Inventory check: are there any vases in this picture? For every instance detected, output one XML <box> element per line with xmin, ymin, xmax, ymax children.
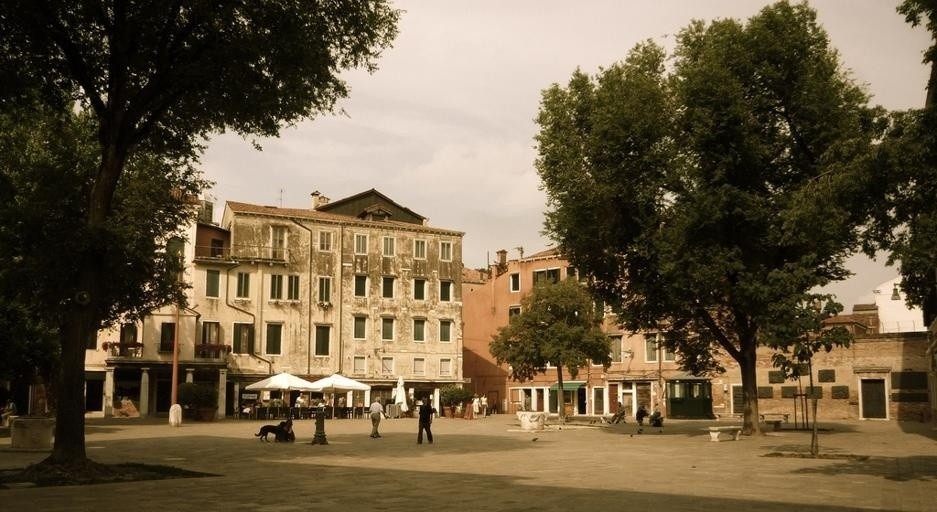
<box><xmin>515</xmin><ymin>409</ymin><xmax>547</xmax><ymax>430</ymax></box>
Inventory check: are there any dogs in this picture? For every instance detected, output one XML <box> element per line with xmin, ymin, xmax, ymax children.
<box><xmin>253</xmin><ymin>421</ymin><xmax>289</xmax><ymax>442</ymax></box>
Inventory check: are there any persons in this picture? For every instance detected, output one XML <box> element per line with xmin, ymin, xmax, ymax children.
<box><xmin>649</xmin><ymin>403</ymin><xmax>661</xmax><ymax>424</ymax></box>
<box><xmin>471</xmin><ymin>392</ymin><xmax>481</xmax><ymax>419</ymax></box>
<box><xmin>294</xmin><ymin>392</ymin><xmax>309</xmax><ymax>408</ymax></box>
<box><xmin>607</xmin><ymin>402</ymin><xmax>625</xmax><ymax>424</ymax></box>
<box><xmin>368</xmin><ymin>396</ymin><xmax>391</xmax><ymax>438</ymax></box>
<box><xmin>491</xmin><ymin>398</ymin><xmax>498</xmax><ymax>415</ymax></box>
<box><xmin>416</xmin><ymin>393</ymin><xmax>434</xmax><ymax>445</ymax></box>
<box><xmin>338</xmin><ymin>394</ymin><xmax>346</xmax><ymax>408</ymax></box>
<box><xmin>0</xmin><ymin>397</ymin><xmax>19</xmax><ymax>421</ymax></box>
<box><xmin>480</xmin><ymin>392</ymin><xmax>489</xmax><ymax>418</ymax></box>
<box><xmin>636</xmin><ymin>405</ymin><xmax>649</xmax><ymax>426</ymax></box>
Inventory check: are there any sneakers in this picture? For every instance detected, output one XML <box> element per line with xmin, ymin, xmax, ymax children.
<box><xmin>370</xmin><ymin>435</ymin><xmax>382</xmax><ymax>438</ymax></box>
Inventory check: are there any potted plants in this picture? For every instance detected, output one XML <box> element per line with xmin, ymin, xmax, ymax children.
<box><xmin>179</xmin><ymin>382</ymin><xmax>217</xmax><ymax>420</ymax></box>
<box><xmin>439</xmin><ymin>384</ymin><xmax>473</xmax><ymax>417</ymax></box>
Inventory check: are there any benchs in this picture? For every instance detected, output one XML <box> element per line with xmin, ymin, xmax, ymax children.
<box><xmin>708</xmin><ymin>426</ymin><xmax>743</xmax><ymax>440</ymax></box>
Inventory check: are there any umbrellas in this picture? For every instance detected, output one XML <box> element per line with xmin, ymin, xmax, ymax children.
<box><xmin>299</xmin><ymin>372</ymin><xmax>373</xmax><ymax>407</ymax></box>
<box><xmin>244</xmin><ymin>371</ymin><xmax>323</xmax><ymax>408</ymax></box>
<box><xmin>395</xmin><ymin>375</ymin><xmax>410</xmax><ymax>413</ymax></box>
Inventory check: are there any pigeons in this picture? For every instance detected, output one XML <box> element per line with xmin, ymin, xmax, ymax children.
<box><xmin>639</xmin><ymin>428</ymin><xmax>643</xmax><ymax>431</ymax></box>
<box><xmin>637</xmin><ymin>430</ymin><xmax>641</xmax><ymax>434</ymax></box>
<box><xmin>531</xmin><ymin>437</ymin><xmax>539</xmax><ymax>442</ymax></box>
